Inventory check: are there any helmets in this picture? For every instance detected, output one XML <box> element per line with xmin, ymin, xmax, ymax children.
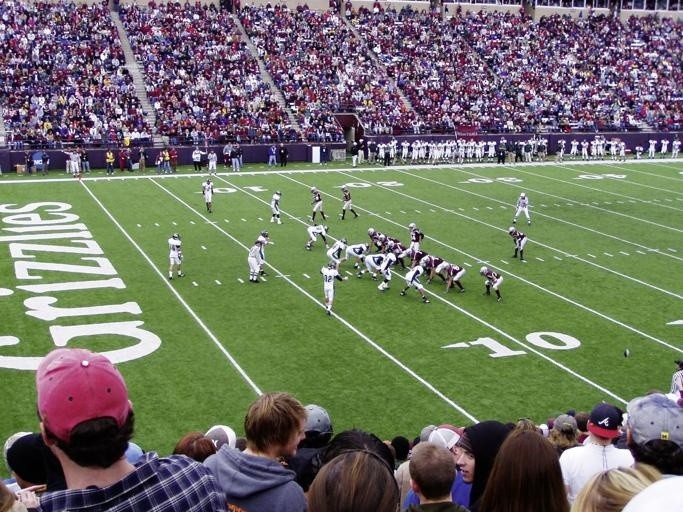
<box><xmin>479</xmin><ymin>266</ymin><xmax>488</xmax><ymax>274</ymax></box>
<box><xmin>255</xmin><ymin>230</ymin><xmax>269</xmax><ymax>248</ymax></box>
<box><xmin>341</xmin><ymin>185</ymin><xmax>347</xmax><ymax>191</ymax></box>
<box><xmin>520</xmin><ymin>193</ymin><xmax>526</xmax><ymax>197</ymax></box>
<box><xmin>311</xmin><ymin>186</ymin><xmax>318</xmax><ymax>194</ymax></box>
<box><xmin>276</xmin><ymin>191</ymin><xmax>282</xmax><ymax>195</ymax></box>
<box><xmin>320</xmin><ymin>221</ymin><xmax>451</xmax><ymax>271</ymax></box>
<box><xmin>207</xmin><ymin>179</ymin><xmax>212</xmax><ymax>183</ymax></box>
<box><xmin>508</xmin><ymin>226</ymin><xmax>516</xmax><ymax>233</ymax></box>
<box><xmin>172</xmin><ymin>233</ymin><xmax>179</xmax><ymax>239</ymax></box>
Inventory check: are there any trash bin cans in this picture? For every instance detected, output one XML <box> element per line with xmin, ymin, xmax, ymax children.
<box><xmin>306</xmin><ymin>144</ymin><xmax>321</xmax><ymax>163</ymax></box>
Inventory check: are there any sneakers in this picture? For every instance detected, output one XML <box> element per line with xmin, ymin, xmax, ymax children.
<box><xmin>270</xmin><ymin>217</ymin><xmax>283</xmax><ymax>225</ymax></box>
<box><xmin>248</xmin><ymin>270</ymin><xmax>267</xmax><ymax>283</ymax></box>
<box><xmin>352</xmin><ymin>260</ymin><xmax>503</xmax><ymax>303</ymax></box>
<box><xmin>324</xmin><ymin>304</ymin><xmax>334</xmax><ymax>315</ymax></box>
<box><xmin>168</xmin><ymin>271</ymin><xmax>185</xmax><ymax>280</ymax></box>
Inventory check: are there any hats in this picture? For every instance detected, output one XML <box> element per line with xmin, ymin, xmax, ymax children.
<box><xmin>627</xmin><ymin>393</ymin><xmax>683</xmax><ymax>456</ymax></box>
<box><xmin>540</xmin><ymin>402</ymin><xmax>623</xmax><ymax>440</ymax></box>
<box><xmin>203</xmin><ymin>425</ymin><xmax>236</xmax><ymax>453</ymax></box>
<box><xmin>419</xmin><ymin>424</ymin><xmax>471</xmax><ymax>455</ymax></box>
<box><xmin>32</xmin><ymin>342</ymin><xmax>133</xmax><ymax>441</ymax></box>
<box><xmin>303</xmin><ymin>404</ymin><xmax>331</xmax><ymax>436</ymax></box>
<box><xmin>3</xmin><ymin>431</ymin><xmax>67</xmax><ymax>492</ymax></box>
